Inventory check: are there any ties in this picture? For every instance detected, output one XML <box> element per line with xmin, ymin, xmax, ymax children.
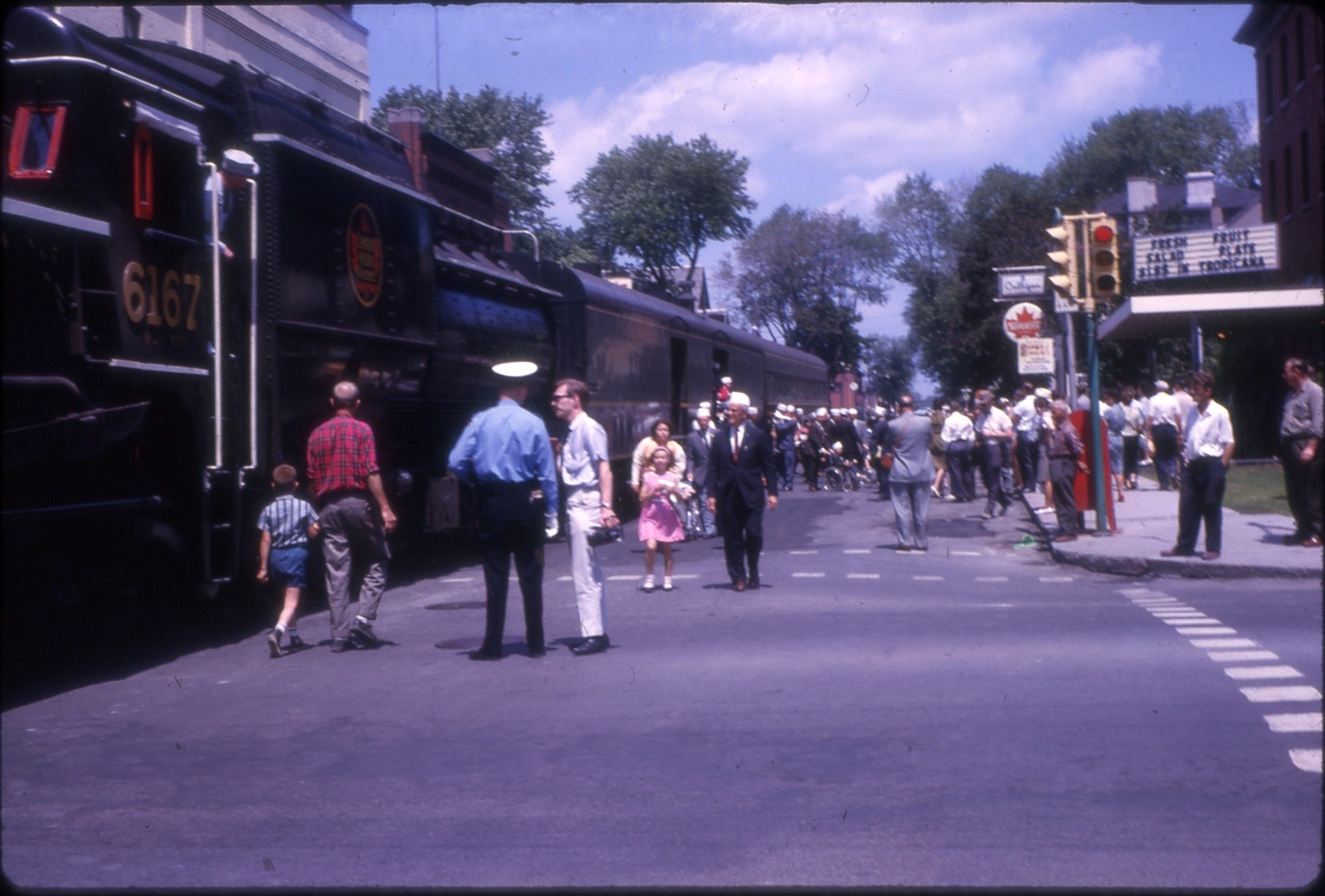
<box><xmin>732</xmin><ymin>423</ymin><xmax>740</xmax><ymax>463</ymax></box>
<box><xmin>559</xmin><ymin>428</ymin><xmax>570</xmax><ymax>445</ymax></box>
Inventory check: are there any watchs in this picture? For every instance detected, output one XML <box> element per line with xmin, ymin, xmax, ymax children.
<box><xmin>599</xmin><ymin>500</ymin><xmax>613</xmax><ymax>509</ymax></box>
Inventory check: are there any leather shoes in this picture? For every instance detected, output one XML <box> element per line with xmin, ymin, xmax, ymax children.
<box><xmin>734</xmin><ymin>581</ymin><xmax>746</xmax><ymax>591</ymax></box>
<box><xmin>1284</xmin><ymin>532</ymin><xmax>1325</xmax><ymax>547</ymax></box>
<box><xmin>529</xmin><ymin>646</ymin><xmax>547</xmax><ymax>658</ymax></box>
<box><xmin>670</xmin><ymin>453</ymin><xmax>1186</xmax><ymax>554</ymax></box>
<box><xmin>643</xmin><ymin>585</ymin><xmax>656</xmax><ymax>593</ymax></box>
<box><xmin>1201</xmin><ymin>550</ymin><xmax>1220</xmax><ymax>561</ymax></box>
<box><xmin>468</xmin><ymin>648</ymin><xmax>501</xmax><ymax>662</ymax></box>
<box><xmin>350</xmin><ymin>618</ymin><xmax>382</xmax><ymax>645</ymax></box>
<box><xmin>662</xmin><ymin>584</ymin><xmax>673</xmax><ymax>591</ymax></box>
<box><xmin>569</xmin><ymin>633</ymin><xmax>610</xmax><ymax>655</ymax></box>
<box><xmin>331</xmin><ymin>638</ymin><xmax>358</xmax><ymax>653</ymax></box>
<box><xmin>748</xmin><ymin>571</ymin><xmax>760</xmax><ymax>588</ymax></box>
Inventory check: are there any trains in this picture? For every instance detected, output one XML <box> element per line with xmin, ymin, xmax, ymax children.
<box><xmin>0</xmin><ymin>0</ymin><xmax>829</xmax><ymax>603</ymax></box>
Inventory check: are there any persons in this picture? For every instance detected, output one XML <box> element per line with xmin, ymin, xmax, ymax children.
<box><xmin>1274</xmin><ymin>358</ymin><xmax>1324</xmax><ymax>547</ymax></box>
<box><xmin>305</xmin><ymin>381</ymin><xmax>399</xmax><ymax>652</ymax></box>
<box><xmin>547</xmin><ymin>378</ymin><xmax>621</xmax><ymax>655</ymax></box>
<box><xmin>257</xmin><ymin>465</ymin><xmax>321</xmax><ymax>657</ymax></box>
<box><xmin>626</xmin><ymin>374</ymin><xmax>1236</xmax><ymax>591</ymax></box>
<box><xmin>446</xmin><ymin>362</ymin><xmax>558</xmax><ymax>658</ymax></box>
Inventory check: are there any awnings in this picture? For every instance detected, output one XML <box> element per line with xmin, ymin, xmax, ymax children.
<box><xmin>1096</xmin><ymin>288</ymin><xmax>1325</xmax><ymax>376</ymax></box>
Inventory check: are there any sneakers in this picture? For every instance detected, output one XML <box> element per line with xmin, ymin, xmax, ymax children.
<box><xmin>289</xmin><ymin>635</ymin><xmax>309</xmax><ymax>654</ymax></box>
<box><xmin>267</xmin><ymin>629</ymin><xmax>284</xmax><ymax>658</ymax></box>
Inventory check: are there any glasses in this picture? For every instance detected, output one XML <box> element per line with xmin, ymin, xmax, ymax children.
<box><xmin>551</xmin><ymin>393</ymin><xmax>572</xmax><ymax>401</ymax></box>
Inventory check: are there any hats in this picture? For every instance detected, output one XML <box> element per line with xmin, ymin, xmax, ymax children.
<box><xmin>1154</xmin><ymin>379</ymin><xmax>1171</xmax><ymax>391</ymax></box>
<box><xmin>645</xmin><ymin>378</ymin><xmax>1066</xmax><ymax>423</ymax></box>
<box><xmin>489</xmin><ymin>361</ymin><xmax>541</xmax><ymax>387</ymax></box>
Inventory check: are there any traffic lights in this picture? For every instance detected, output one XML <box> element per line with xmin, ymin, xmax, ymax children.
<box><xmin>1044</xmin><ymin>224</ymin><xmax>1077</xmax><ymax>305</ymax></box>
<box><xmin>1089</xmin><ymin>219</ymin><xmax>1120</xmax><ymax>298</ymax></box>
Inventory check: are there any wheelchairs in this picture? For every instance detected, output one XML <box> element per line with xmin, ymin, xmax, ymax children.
<box><xmin>824</xmin><ymin>452</ymin><xmax>858</xmax><ymax>493</ymax></box>
